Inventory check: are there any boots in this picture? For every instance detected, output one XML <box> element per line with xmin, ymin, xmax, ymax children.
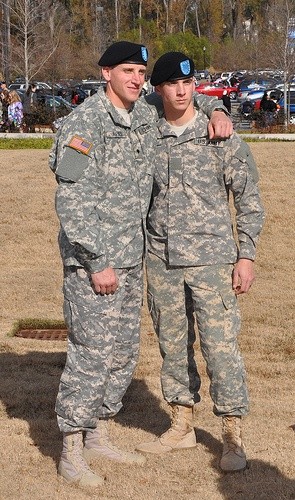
<box><xmin>84</xmin><ymin>419</ymin><xmax>147</xmax><ymax>466</ymax></box>
<box><xmin>57</xmin><ymin>430</ymin><xmax>105</xmax><ymax>490</ymax></box>
<box><xmin>134</xmin><ymin>404</ymin><xmax>197</xmax><ymax>454</ymax></box>
<box><xmin>221</xmin><ymin>415</ymin><xmax>247</xmax><ymax>470</ymax></box>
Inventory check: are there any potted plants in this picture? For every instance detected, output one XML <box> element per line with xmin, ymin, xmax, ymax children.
<box><xmin>22</xmin><ymin>103</ymin><xmax>57</xmax><ymax>133</ymax></box>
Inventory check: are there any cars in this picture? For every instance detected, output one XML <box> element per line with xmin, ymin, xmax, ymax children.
<box><xmin>0</xmin><ymin>77</ymin><xmax>106</xmax><ymax>125</ymax></box>
<box><xmin>193</xmin><ymin>66</ymin><xmax>295</xmax><ymax>125</ymax></box>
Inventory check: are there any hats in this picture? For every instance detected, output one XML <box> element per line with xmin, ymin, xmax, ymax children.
<box><xmin>150</xmin><ymin>52</ymin><xmax>196</xmax><ymax>86</ymax></box>
<box><xmin>97</xmin><ymin>40</ymin><xmax>148</xmax><ymax>67</ymax></box>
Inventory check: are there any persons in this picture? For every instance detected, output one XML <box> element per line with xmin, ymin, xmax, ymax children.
<box><xmin>49</xmin><ymin>41</ymin><xmax>232</xmax><ymax>488</ymax></box>
<box><xmin>0</xmin><ymin>79</ymin><xmax>38</xmax><ymax>133</ymax></box>
<box><xmin>134</xmin><ymin>53</ymin><xmax>264</xmax><ymax>471</ymax></box>
